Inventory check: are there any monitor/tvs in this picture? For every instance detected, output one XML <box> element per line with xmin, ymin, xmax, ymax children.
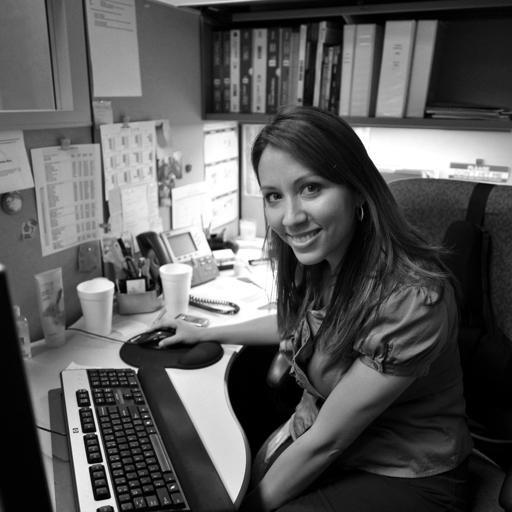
<box><xmin>0</xmin><ymin>266</ymin><xmax>53</xmax><ymax>512</ymax></box>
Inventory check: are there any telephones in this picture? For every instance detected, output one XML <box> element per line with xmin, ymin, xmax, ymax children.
<box><xmin>136</xmin><ymin>227</ymin><xmax>219</xmax><ymax>288</ymax></box>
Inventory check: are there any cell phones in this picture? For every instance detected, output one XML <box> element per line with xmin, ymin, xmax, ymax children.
<box><xmin>175</xmin><ymin>313</ymin><xmax>208</xmax><ymax>327</ymax></box>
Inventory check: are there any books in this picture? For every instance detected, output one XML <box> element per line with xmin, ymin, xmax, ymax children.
<box><xmin>209</xmin><ymin>17</ymin><xmax>439</xmax><ymax>119</ymax></box>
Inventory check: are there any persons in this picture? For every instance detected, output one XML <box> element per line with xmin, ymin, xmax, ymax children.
<box><xmin>143</xmin><ymin>104</ymin><xmax>482</xmax><ymax>509</ymax></box>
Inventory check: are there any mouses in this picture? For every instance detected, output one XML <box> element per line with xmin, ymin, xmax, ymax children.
<box><xmin>138</xmin><ymin>327</ymin><xmax>175</xmax><ymax>347</ymax></box>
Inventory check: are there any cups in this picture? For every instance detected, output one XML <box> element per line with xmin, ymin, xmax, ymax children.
<box><xmin>75</xmin><ymin>277</ymin><xmax>116</xmax><ymax>341</ymax></box>
<box><xmin>155</xmin><ymin>263</ymin><xmax>196</xmax><ymax>319</ymax></box>
<box><xmin>240</xmin><ymin>217</ymin><xmax>257</xmax><ymax>238</ymax></box>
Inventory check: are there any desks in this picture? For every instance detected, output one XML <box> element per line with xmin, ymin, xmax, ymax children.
<box><xmin>21</xmin><ymin>235</ymin><xmax>279</xmax><ymax>512</ymax></box>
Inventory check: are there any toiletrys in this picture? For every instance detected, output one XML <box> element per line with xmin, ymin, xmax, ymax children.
<box><xmin>34</xmin><ymin>266</ymin><xmax>66</xmax><ymax>349</ymax></box>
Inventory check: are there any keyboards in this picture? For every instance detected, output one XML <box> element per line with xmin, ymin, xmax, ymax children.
<box><xmin>60</xmin><ymin>369</ymin><xmax>190</xmax><ymax>512</ymax></box>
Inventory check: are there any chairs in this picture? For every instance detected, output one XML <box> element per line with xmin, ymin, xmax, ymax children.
<box><xmin>381</xmin><ymin>178</ymin><xmax>512</xmax><ymax>510</ymax></box>
